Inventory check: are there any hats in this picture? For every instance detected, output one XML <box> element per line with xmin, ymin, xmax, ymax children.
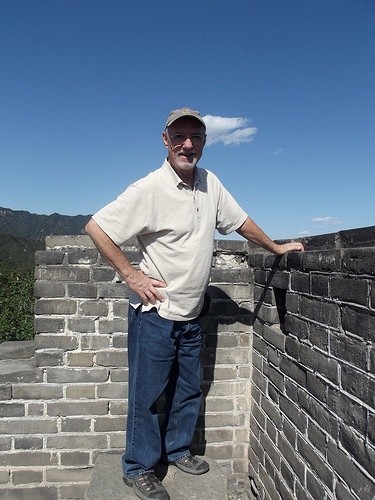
<box><xmin>163</xmin><ymin>107</ymin><xmax>208</xmax><ymax>130</ymax></box>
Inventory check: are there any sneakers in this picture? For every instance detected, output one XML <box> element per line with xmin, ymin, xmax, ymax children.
<box><xmin>169</xmin><ymin>453</ymin><xmax>210</xmax><ymax>475</ymax></box>
<box><xmin>122</xmin><ymin>469</ymin><xmax>173</xmax><ymax>500</ymax></box>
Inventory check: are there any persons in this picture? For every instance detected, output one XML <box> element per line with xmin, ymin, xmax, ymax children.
<box><xmin>84</xmin><ymin>107</ymin><xmax>305</xmax><ymax>500</ymax></box>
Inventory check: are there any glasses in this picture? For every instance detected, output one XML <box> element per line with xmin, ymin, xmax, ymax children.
<box><xmin>167</xmin><ymin>132</ymin><xmax>207</xmax><ymax>144</ymax></box>
